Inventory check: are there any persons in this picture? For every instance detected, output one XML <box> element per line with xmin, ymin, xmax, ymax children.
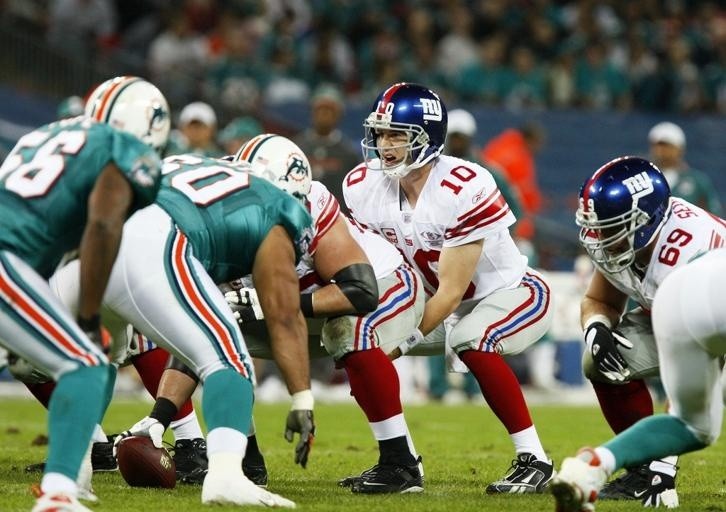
<box><xmin>0</xmin><ymin>74</ymin><xmax>169</xmax><ymax>511</ymax></box>
<box><xmin>1</xmin><ymin>1</ymin><xmax>725</xmax><ymax>404</ymax></box>
<box><xmin>551</xmin><ymin>155</ymin><xmax>725</xmax><ymax>511</ymax></box>
<box><xmin>46</xmin><ymin>84</ymin><xmax>553</xmax><ymax>508</ymax></box>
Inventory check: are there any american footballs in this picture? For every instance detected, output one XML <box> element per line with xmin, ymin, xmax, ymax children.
<box><xmin>116</xmin><ymin>436</ymin><xmax>176</xmax><ymax>487</ymax></box>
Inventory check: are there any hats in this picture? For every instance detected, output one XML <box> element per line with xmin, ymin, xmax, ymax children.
<box><xmin>176</xmin><ymin>101</ymin><xmax>217</xmax><ymax>128</ymax></box>
<box><xmin>445</xmin><ymin>108</ymin><xmax>476</xmax><ymax>138</ymax></box>
<box><xmin>648</xmin><ymin>121</ymin><xmax>686</xmax><ymax>149</ymax></box>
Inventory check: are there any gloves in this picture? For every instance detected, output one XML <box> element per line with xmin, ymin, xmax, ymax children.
<box><xmin>76</xmin><ymin>312</ymin><xmax>104</xmax><ymax>354</ymax></box>
<box><xmin>583</xmin><ymin>321</ymin><xmax>633</xmax><ymax>382</ymax></box>
<box><xmin>113</xmin><ymin>416</ymin><xmax>165</xmax><ymax>456</ymax></box>
<box><xmin>284</xmin><ymin>389</ymin><xmax>315</xmax><ymax>467</ymax></box>
<box><xmin>632</xmin><ymin>460</ymin><xmax>680</xmax><ymax>509</ymax></box>
<box><xmin>223</xmin><ymin>286</ymin><xmax>265</xmax><ymax>325</ymax></box>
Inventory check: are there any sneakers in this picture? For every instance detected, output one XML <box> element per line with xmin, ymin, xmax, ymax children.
<box><xmin>485</xmin><ymin>453</ymin><xmax>557</xmax><ymax>494</ymax></box>
<box><xmin>23</xmin><ymin>435</ymin><xmax>297</xmax><ymax>512</ymax></box>
<box><xmin>350</xmin><ymin>454</ymin><xmax>424</xmax><ymax>495</ymax></box>
<box><xmin>597</xmin><ymin>465</ymin><xmax>648</xmax><ymax>500</ymax></box>
<box><xmin>548</xmin><ymin>447</ymin><xmax>608</xmax><ymax>512</ymax></box>
<box><xmin>336</xmin><ymin>452</ymin><xmax>425</xmax><ymax>488</ymax></box>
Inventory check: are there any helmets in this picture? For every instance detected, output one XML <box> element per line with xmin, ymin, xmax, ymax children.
<box><xmin>83</xmin><ymin>74</ymin><xmax>171</xmax><ymax>157</ymax></box>
<box><xmin>230</xmin><ymin>132</ymin><xmax>312</xmax><ymax>198</ymax></box>
<box><xmin>359</xmin><ymin>82</ymin><xmax>448</xmax><ymax>182</ymax></box>
<box><xmin>574</xmin><ymin>155</ymin><xmax>672</xmax><ymax>275</ymax></box>
